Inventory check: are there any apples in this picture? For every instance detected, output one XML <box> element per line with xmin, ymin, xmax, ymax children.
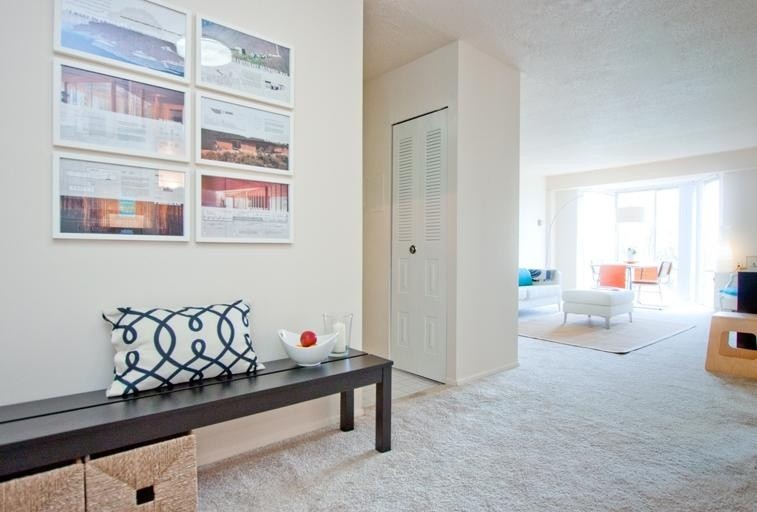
<box><xmin>300</xmin><ymin>332</ymin><xmax>316</xmax><ymax>347</ymax></box>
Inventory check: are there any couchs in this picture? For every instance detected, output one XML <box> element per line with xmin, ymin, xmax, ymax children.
<box><xmin>517</xmin><ymin>265</ymin><xmax>567</xmax><ymax>311</ymax></box>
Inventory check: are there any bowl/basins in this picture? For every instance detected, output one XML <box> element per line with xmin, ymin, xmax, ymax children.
<box><xmin>278</xmin><ymin>329</ymin><xmax>339</xmax><ymax>366</ymax></box>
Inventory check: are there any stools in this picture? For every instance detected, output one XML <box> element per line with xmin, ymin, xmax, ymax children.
<box><xmin>704</xmin><ymin>310</ymin><xmax>757</xmax><ymax>380</ymax></box>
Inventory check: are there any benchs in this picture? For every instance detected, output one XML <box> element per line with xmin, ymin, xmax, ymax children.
<box><xmin>0</xmin><ymin>340</ymin><xmax>394</xmax><ymax>479</ymax></box>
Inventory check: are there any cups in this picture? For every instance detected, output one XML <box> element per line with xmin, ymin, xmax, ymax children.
<box><xmin>321</xmin><ymin>312</ymin><xmax>353</xmax><ymax>357</ymax></box>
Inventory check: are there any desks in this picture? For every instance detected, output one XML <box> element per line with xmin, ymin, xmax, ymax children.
<box><xmin>561</xmin><ymin>282</ymin><xmax>636</xmax><ymax>330</ymax></box>
<box><xmin>736</xmin><ymin>270</ymin><xmax>757</xmax><ymax>312</ymax></box>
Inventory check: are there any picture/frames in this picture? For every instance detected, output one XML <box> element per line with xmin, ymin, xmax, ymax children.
<box><xmin>52</xmin><ymin>59</ymin><xmax>192</xmax><ymax>164</ymax></box>
<box><xmin>194</xmin><ymin>91</ymin><xmax>293</xmax><ymax>175</ymax></box>
<box><xmin>51</xmin><ymin>152</ymin><xmax>190</xmax><ymax>242</ymax></box>
<box><xmin>194</xmin><ymin>170</ymin><xmax>293</xmax><ymax>244</ymax></box>
<box><xmin>194</xmin><ymin>14</ymin><xmax>293</xmax><ymax>108</ymax></box>
<box><xmin>50</xmin><ymin>1</ymin><xmax>192</xmax><ymax>85</ymax></box>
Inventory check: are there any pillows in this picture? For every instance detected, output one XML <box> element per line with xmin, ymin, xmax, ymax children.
<box><xmin>517</xmin><ymin>268</ymin><xmax>557</xmax><ymax>288</ymax></box>
<box><xmin>100</xmin><ymin>299</ymin><xmax>267</xmax><ymax>398</ymax></box>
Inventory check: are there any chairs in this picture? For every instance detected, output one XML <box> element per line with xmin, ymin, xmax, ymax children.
<box><xmin>586</xmin><ymin>257</ymin><xmax>673</xmax><ymax>311</ymax></box>
